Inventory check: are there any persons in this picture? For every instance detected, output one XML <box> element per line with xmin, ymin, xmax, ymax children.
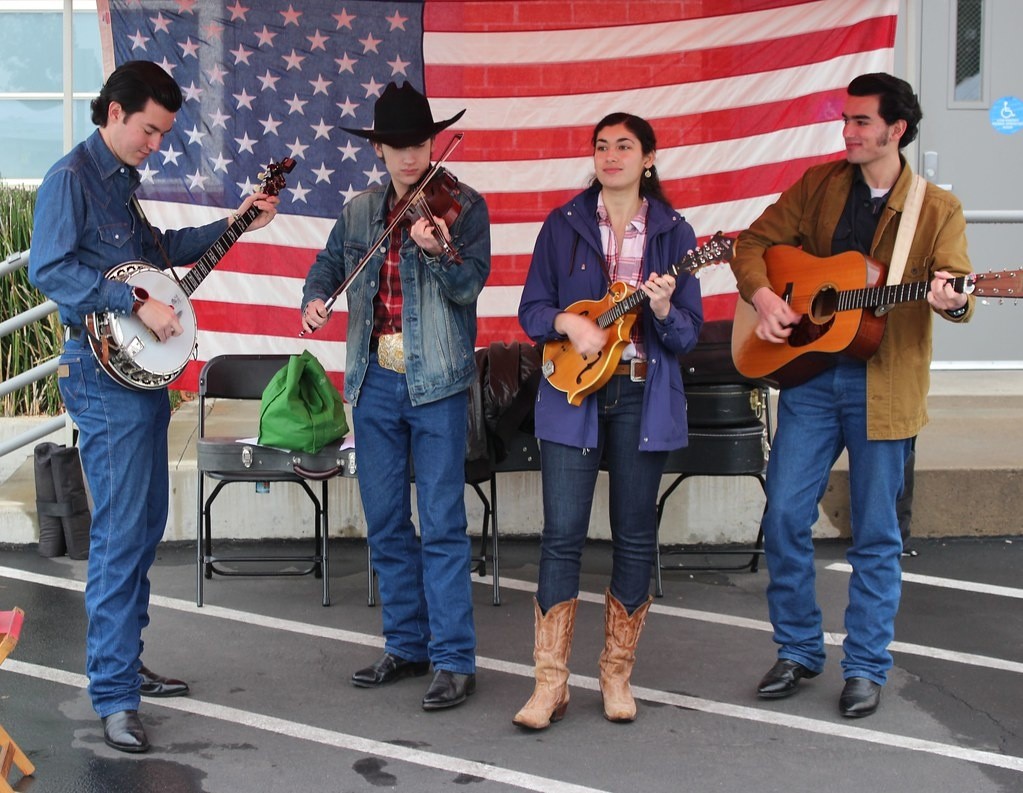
<box><xmin>512</xmin><ymin>111</ymin><xmax>704</xmax><ymax>730</ymax></box>
<box><xmin>27</xmin><ymin>60</ymin><xmax>281</xmax><ymax>751</ymax></box>
<box><xmin>299</xmin><ymin>80</ymin><xmax>492</xmax><ymax>710</ymax></box>
<box><xmin>726</xmin><ymin>72</ymin><xmax>977</xmax><ymax>719</ymax></box>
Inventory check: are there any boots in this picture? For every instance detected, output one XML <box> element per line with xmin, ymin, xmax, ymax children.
<box><xmin>599</xmin><ymin>584</ymin><xmax>653</xmax><ymax>722</ymax></box>
<box><xmin>513</xmin><ymin>589</ymin><xmax>578</xmax><ymax>730</ymax></box>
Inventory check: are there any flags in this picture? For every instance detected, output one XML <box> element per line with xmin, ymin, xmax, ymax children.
<box><xmin>108</xmin><ymin>0</ymin><xmax>900</xmax><ymax>403</ymax></box>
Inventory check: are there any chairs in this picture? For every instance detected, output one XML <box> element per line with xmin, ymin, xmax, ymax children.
<box><xmin>345</xmin><ymin>319</ymin><xmax>774</xmax><ymax>607</ymax></box>
<box><xmin>196</xmin><ymin>354</ymin><xmax>330</xmax><ymax>607</ymax></box>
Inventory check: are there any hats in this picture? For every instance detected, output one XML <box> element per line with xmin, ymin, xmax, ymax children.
<box><xmin>339</xmin><ymin>80</ymin><xmax>468</xmax><ymax>150</ymax></box>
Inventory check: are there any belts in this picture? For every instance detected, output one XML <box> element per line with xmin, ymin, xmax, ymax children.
<box><xmin>369</xmin><ymin>333</ymin><xmax>409</xmax><ymax>374</ymax></box>
<box><xmin>609</xmin><ymin>360</ymin><xmax>652</xmax><ymax>382</ymax></box>
<box><xmin>68</xmin><ymin>326</ymin><xmax>92</xmax><ymax>346</ymax></box>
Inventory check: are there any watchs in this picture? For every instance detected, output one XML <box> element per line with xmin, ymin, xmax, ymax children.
<box><xmin>132</xmin><ymin>287</ymin><xmax>149</xmax><ymax>313</ymax></box>
<box><xmin>946</xmin><ymin>306</ymin><xmax>967</xmax><ymax>317</ymax></box>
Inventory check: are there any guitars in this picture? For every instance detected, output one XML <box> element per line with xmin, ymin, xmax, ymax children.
<box><xmin>85</xmin><ymin>156</ymin><xmax>297</xmax><ymax>391</ymax></box>
<box><xmin>542</xmin><ymin>230</ymin><xmax>738</xmax><ymax>407</ymax></box>
<box><xmin>731</xmin><ymin>244</ymin><xmax>1023</xmax><ymax>390</ymax></box>
<box><xmin>385</xmin><ymin>165</ymin><xmax>464</xmax><ymax>267</ymax></box>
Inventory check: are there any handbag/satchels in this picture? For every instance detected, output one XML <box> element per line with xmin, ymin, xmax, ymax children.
<box><xmin>256</xmin><ymin>350</ymin><xmax>349</xmax><ymax>455</ymax></box>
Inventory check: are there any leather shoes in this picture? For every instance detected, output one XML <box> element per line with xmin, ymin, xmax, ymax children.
<box><xmin>754</xmin><ymin>657</ymin><xmax>820</xmax><ymax>699</ymax></box>
<box><xmin>135</xmin><ymin>664</ymin><xmax>190</xmax><ymax>696</ymax></box>
<box><xmin>838</xmin><ymin>675</ymin><xmax>882</xmax><ymax>718</ymax></box>
<box><xmin>348</xmin><ymin>651</ymin><xmax>431</xmax><ymax>688</ymax></box>
<box><xmin>101</xmin><ymin>710</ymin><xmax>150</xmax><ymax>751</ymax></box>
<box><xmin>421</xmin><ymin>667</ymin><xmax>478</xmax><ymax>710</ymax></box>
